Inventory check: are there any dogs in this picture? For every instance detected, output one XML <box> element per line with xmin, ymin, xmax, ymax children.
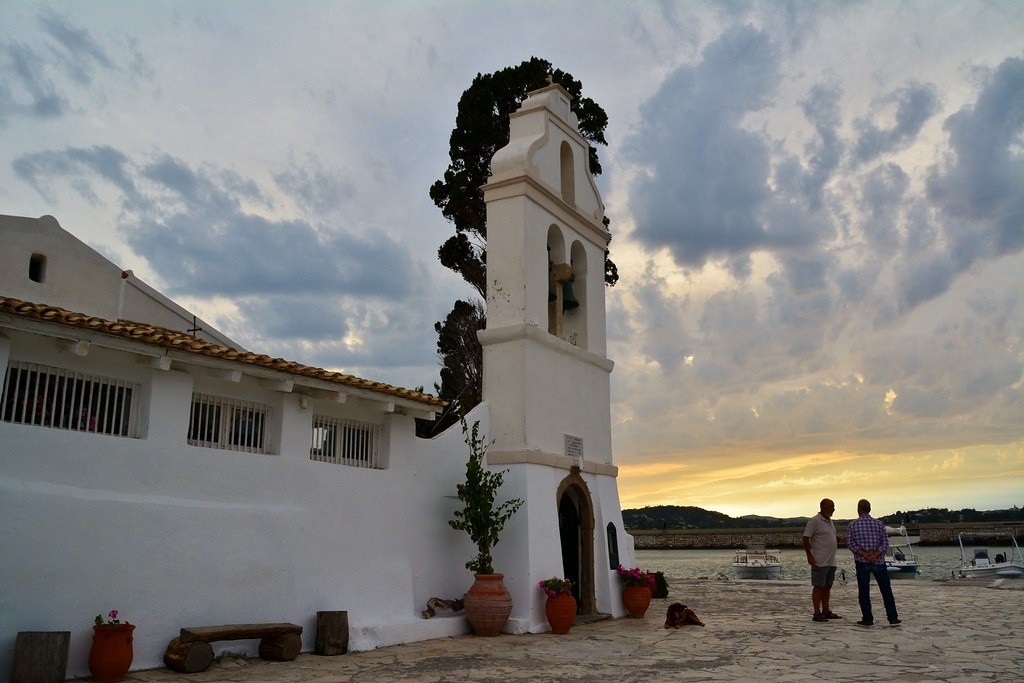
<box><xmin>663</xmin><ymin>602</ymin><xmax>704</xmax><ymax>629</ymax></box>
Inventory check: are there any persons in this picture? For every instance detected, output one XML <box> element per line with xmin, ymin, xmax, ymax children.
<box><xmin>847</xmin><ymin>499</ymin><xmax>902</xmax><ymax>626</ymax></box>
<box><xmin>802</xmin><ymin>499</ymin><xmax>842</xmax><ymax>622</ymax></box>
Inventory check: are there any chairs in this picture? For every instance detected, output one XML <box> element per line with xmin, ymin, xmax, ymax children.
<box><xmin>971</xmin><ymin>552</ymin><xmax>991</xmax><ymax>565</ymax></box>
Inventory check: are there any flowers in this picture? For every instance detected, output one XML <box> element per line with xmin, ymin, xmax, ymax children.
<box><xmin>616</xmin><ymin>563</ymin><xmax>655</xmax><ymax>590</ymax></box>
<box><xmin>94</xmin><ymin>610</ymin><xmax>130</xmax><ymax>625</ymax></box>
<box><xmin>537</xmin><ymin>576</ymin><xmax>575</xmax><ymax>597</ymax></box>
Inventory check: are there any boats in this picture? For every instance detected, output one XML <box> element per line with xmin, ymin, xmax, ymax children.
<box><xmin>952</xmin><ymin>528</ymin><xmax>1024</xmax><ymax>579</ymax></box>
<box><xmin>731</xmin><ymin>543</ymin><xmax>784</xmax><ymax>581</ymax></box>
<box><xmin>883</xmin><ymin>525</ymin><xmax>922</xmax><ymax>575</ymax></box>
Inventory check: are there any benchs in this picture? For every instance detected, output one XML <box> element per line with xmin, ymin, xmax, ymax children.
<box><xmin>163</xmin><ymin>623</ymin><xmax>303</xmax><ymax>674</ymax></box>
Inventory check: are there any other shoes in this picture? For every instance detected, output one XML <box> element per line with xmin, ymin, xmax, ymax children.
<box><xmin>857</xmin><ymin>617</ymin><xmax>874</xmax><ymax>626</ymax></box>
<box><xmin>821</xmin><ymin>610</ymin><xmax>842</xmax><ymax>619</ymax></box>
<box><xmin>812</xmin><ymin>613</ymin><xmax>828</xmax><ymax>622</ymax></box>
<box><xmin>887</xmin><ymin>618</ymin><xmax>902</xmax><ymax>626</ymax></box>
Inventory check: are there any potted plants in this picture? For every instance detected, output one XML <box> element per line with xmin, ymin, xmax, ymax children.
<box><xmin>448</xmin><ymin>396</ymin><xmax>525</xmax><ymax>635</ymax></box>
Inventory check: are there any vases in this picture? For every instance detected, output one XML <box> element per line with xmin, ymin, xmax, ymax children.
<box><xmin>624</xmin><ymin>584</ymin><xmax>652</xmax><ymax>619</ymax></box>
<box><xmin>89</xmin><ymin>624</ymin><xmax>135</xmax><ymax>683</ymax></box>
<box><xmin>545</xmin><ymin>590</ymin><xmax>578</xmax><ymax>634</ymax></box>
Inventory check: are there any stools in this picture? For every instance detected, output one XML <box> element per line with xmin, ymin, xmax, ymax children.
<box><xmin>314</xmin><ymin>611</ymin><xmax>349</xmax><ymax>656</ymax></box>
<box><xmin>11</xmin><ymin>630</ymin><xmax>70</xmax><ymax>683</ymax></box>
